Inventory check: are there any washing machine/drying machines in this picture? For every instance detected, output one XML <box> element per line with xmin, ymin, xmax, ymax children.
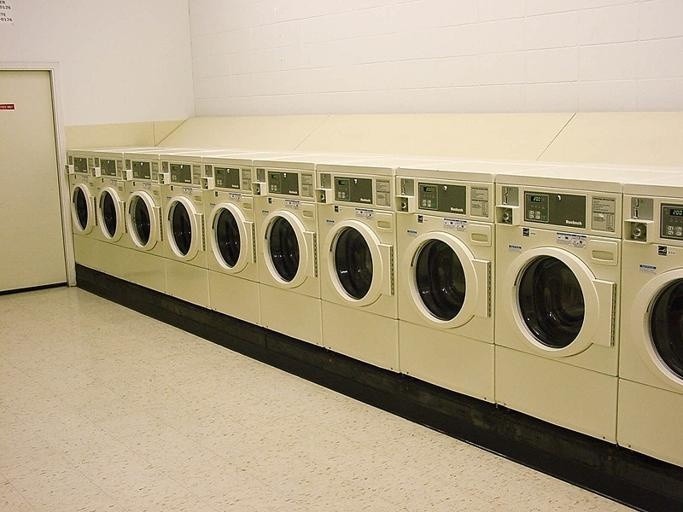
<box><xmin>66</xmin><ymin>117</ymin><xmax>683</xmax><ymax>468</ymax></box>
